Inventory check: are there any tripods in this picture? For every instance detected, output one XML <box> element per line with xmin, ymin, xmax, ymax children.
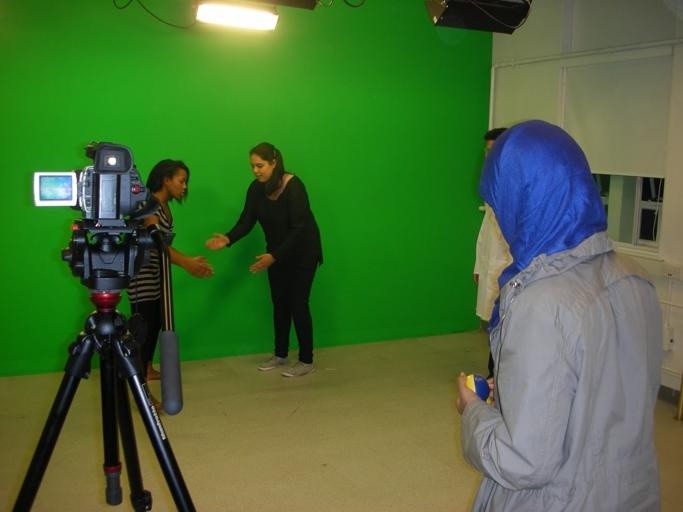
<box><xmin>13</xmin><ymin>310</ymin><xmax>197</xmax><ymax>512</ymax></box>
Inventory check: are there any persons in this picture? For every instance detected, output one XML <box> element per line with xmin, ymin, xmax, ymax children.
<box><xmin>469</xmin><ymin>127</ymin><xmax>514</xmax><ymax>391</ymax></box>
<box><xmin>456</xmin><ymin>120</ymin><xmax>665</xmax><ymax>512</ymax></box>
<box><xmin>122</xmin><ymin>158</ymin><xmax>215</xmax><ymax>413</ymax></box>
<box><xmin>205</xmin><ymin>141</ymin><xmax>323</xmax><ymax>377</ymax></box>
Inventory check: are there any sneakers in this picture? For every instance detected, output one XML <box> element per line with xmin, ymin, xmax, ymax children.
<box><xmin>258</xmin><ymin>355</ymin><xmax>288</xmax><ymax>370</ymax></box>
<box><xmin>281</xmin><ymin>361</ymin><xmax>314</xmax><ymax>376</ymax></box>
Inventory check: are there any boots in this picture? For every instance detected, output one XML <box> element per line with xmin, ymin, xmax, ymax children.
<box><xmin>146</xmin><ymin>362</ymin><xmax>161</xmax><ymax>380</ymax></box>
<box><xmin>148</xmin><ymin>390</ymin><xmax>161</xmax><ymax>410</ymax></box>
